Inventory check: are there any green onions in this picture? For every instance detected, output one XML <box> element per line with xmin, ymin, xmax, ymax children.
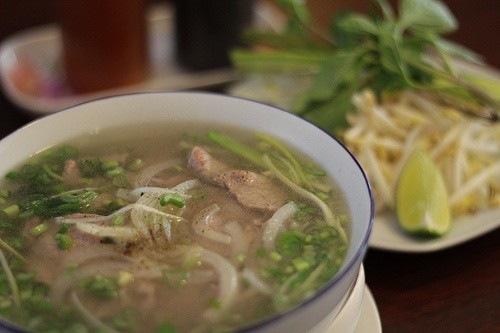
<box><xmin>0</xmin><ymin>119</ymin><xmax>352</xmax><ymax>333</ymax></box>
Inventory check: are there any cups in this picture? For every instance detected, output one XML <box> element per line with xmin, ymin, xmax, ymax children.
<box><xmin>170</xmin><ymin>0</ymin><xmax>253</xmax><ymax>73</ymax></box>
<box><xmin>57</xmin><ymin>0</ymin><xmax>150</xmax><ymax>97</ymax></box>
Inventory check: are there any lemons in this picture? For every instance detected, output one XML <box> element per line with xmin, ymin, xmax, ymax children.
<box><xmin>395</xmin><ymin>147</ymin><xmax>453</xmax><ymax>241</ymax></box>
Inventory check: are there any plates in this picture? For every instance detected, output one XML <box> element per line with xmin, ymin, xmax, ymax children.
<box><xmin>1</xmin><ymin>2</ymin><xmax>291</xmax><ymax>110</ymax></box>
<box><xmin>226</xmin><ymin>44</ymin><xmax>500</xmax><ymax>252</ymax></box>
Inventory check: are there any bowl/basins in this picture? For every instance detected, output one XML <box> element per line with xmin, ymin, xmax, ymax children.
<box><xmin>0</xmin><ymin>91</ymin><xmax>382</xmax><ymax>333</ymax></box>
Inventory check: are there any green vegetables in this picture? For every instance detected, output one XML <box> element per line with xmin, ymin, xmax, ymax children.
<box><xmin>231</xmin><ymin>0</ymin><xmax>500</xmax><ymax>214</ymax></box>
